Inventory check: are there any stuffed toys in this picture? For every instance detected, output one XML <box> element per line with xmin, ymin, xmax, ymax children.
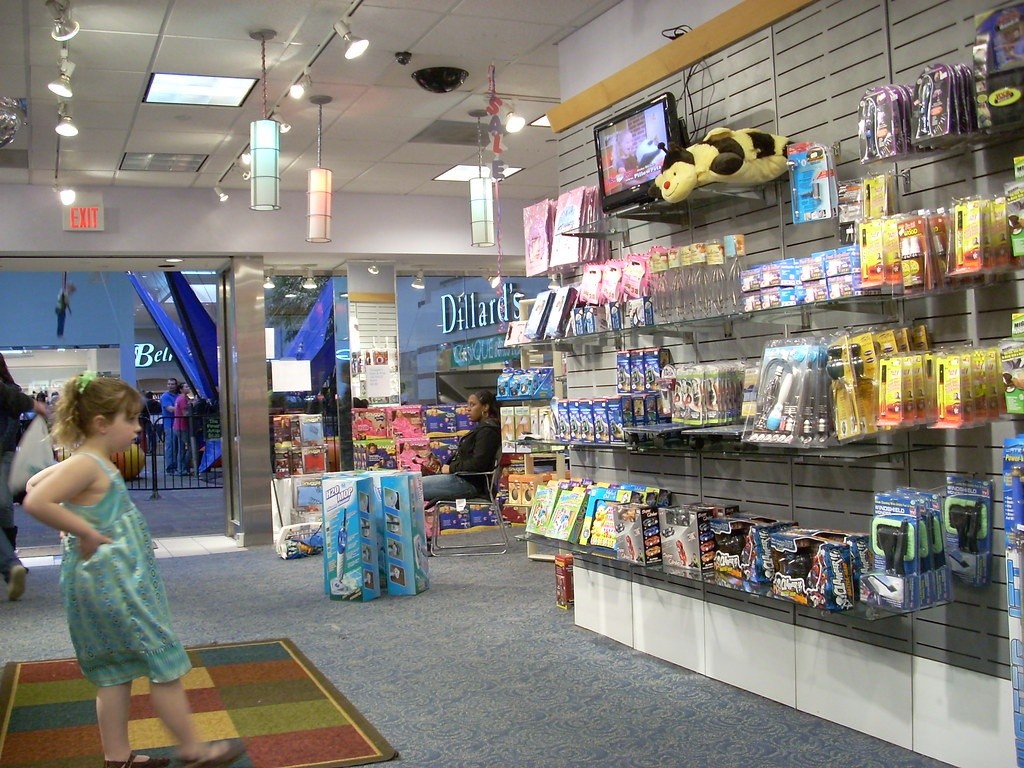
<box><xmin>635</xmin><ymin>140</ymin><xmax>667</xmax><ymax>172</ymax></box>
<box><xmin>648</xmin><ymin>126</ymin><xmax>795</xmax><ymax>204</ymax></box>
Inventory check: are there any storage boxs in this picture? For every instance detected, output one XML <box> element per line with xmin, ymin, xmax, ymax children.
<box><xmin>272</xmin><ymin>243</ymin><xmax>872</xmax><ymax>611</ymax></box>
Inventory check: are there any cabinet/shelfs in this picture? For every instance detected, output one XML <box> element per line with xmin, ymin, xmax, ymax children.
<box><xmin>500</xmin><ymin>287</ymin><xmax>923</xmax><ymax>459</ymax></box>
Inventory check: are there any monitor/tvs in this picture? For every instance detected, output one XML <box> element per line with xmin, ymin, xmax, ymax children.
<box><xmin>593</xmin><ymin>93</ymin><xmax>679</xmax><ymax>213</ymax></box>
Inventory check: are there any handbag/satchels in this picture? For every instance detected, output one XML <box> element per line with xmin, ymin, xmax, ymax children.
<box><xmin>150</xmin><ymin>415</ymin><xmax>164</xmax><ymax>432</ymax></box>
<box><xmin>6</xmin><ymin>413</ymin><xmax>54</xmax><ymax>495</ymax></box>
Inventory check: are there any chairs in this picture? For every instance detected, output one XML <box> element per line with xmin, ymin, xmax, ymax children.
<box><xmin>432</xmin><ymin>448</ymin><xmax>508</xmax><ymax>554</ymax></box>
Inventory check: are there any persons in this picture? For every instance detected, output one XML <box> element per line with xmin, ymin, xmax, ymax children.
<box><xmin>389</xmin><ymin>410</ymin><xmax>422</xmax><ymax>438</ymax></box>
<box><xmin>353</xmin><ymin>411</ymin><xmax>385</xmax><ymax>437</ymax></box>
<box><xmin>172</xmin><ymin>382</ymin><xmax>191</xmax><ymax>475</ymax></box>
<box><xmin>143</xmin><ymin>392</ymin><xmax>162</xmax><ymax>454</ymax></box>
<box><xmin>421</xmin><ymin>389</ymin><xmax>502</xmax><ymax>556</ymax></box>
<box><xmin>368</xmin><ymin>443</ymin><xmax>396</xmax><ymax>469</ymax></box>
<box><xmin>20</xmin><ymin>390</ymin><xmax>62</xmax><ymax>419</ymax></box>
<box><xmin>159</xmin><ymin>377</ymin><xmax>179</xmax><ymax>473</ymax></box>
<box><xmin>399</xmin><ymin>443</ymin><xmax>429</xmax><ymax>476</ymax></box>
<box><xmin>22</xmin><ymin>371</ymin><xmax>247</xmax><ymax>768</ymax></box>
<box><xmin>612</xmin><ymin>129</ymin><xmax>650</xmax><ymax>191</ymax></box>
<box><xmin>0</xmin><ymin>353</ymin><xmax>49</xmax><ymax>601</ymax></box>
<box><xmin>54</xmin><ymin>285</ymin><xmax>76</xmax><ymax>338</ymax></box>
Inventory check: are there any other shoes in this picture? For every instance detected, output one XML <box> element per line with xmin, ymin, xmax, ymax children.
<box><xmin>103</xmin><ymin>751</ymin><xmax>171</xmax><ymax>768</ymax></box>
<box><xmin>181</xmin><ymin>741</ymin><xmax>248</xmax><ymax>768</ymax></box>
<box><xmin>145</xmin><ymin>450</ymin><xmax>152</xmax><ymax>456</ymax></box>
<box><xmin>3</xmin><ymin>556</ymin><xmax>26</xmax><ymax>600</ymax></box>
<box><xmin>166</xmin><ymin>470</ymin><xmax>179</xmax><ymax>475</ymax></box>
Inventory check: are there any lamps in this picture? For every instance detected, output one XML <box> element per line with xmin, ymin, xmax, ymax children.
<box><xmin>46</xmin><ymin>0</ymin><xmax>79</xmax><ymax>41</ymax></box>
<box><xmin>233</xmin><ymin>162</ymin><xmax>250</xmax><ymax>181</ymax></box>
<box><xmin>468</xmin><ymin>109</ymin><xmax>495</xmax><ymax>247</ymax></box>
<box><xmin>214</xmin><ymin>186</ymin><xmax>228</xmax><ymax>201</ymax></box>
<box><xmin>52</xmin><ymin>187</ymin><xmax>75</xmax><ymax>205</ymax></box>
<box><xmin>498</xmin><ymin>101</ymin><xmax>526</xmax><ymax>134</ymax></box>
<box><xmin>336</xmin><ymin>23</ymin><xmax>369</xmax><ymax>59</ymax></box>
<box><xmin>290</xmin><ymin>68</ymin><xmax>313</xmax><ymax>99</ymax></box>
<box><xmin>249</xmin><ymin>29</ymin><xmax>282</xmax><ymax>210</ymax></box>
<box><xmin>48</xmin><ymin>50</ymin><xmax>75</xmax><ymax>98</ymax></box>
<box><xmin>305</xmin><ymin>97</ymin><xmax>335</xmax><ymax>243</ymax></box>
<box><xmin>55</xmin><ymin>103</ymin><xmax>79</xmax><ymax>136</ymax></box>
<box><xmin>273</xmin><ymin>114</ymin><xmax>291</xmax><ymax>134</ymax></box>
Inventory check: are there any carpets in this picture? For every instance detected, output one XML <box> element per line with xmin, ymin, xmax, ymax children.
<box><xmin>0</xmin><ymin>637</ymin><xmax>396</xmax><ymax>768</ymax></box>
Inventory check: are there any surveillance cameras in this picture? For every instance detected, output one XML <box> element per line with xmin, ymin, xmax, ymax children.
<box><xmin>395</xmin><ymin>52</ymin><xmax>411</xmax><ymax>65</ymax></box>
